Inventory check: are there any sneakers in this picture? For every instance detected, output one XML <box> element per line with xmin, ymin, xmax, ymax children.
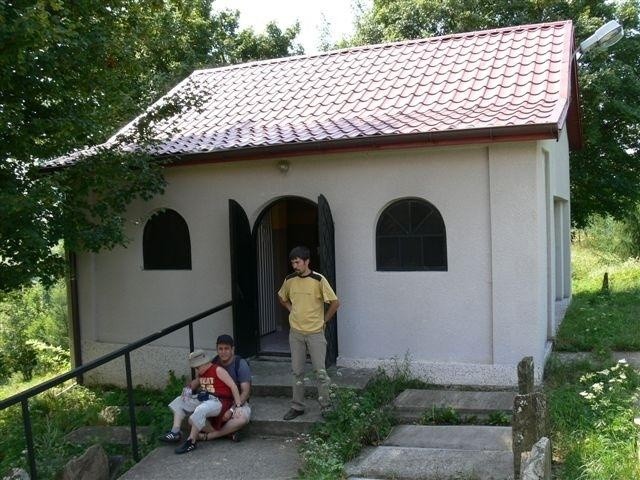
<box><xmin>158</xmin><ymin>432</ymin><xmax>181</xmax><ymax>442</ymax></box>
<box><xmin>321</xmin><ymin>406</ymin><xmax>334</xmax><ymax>416</ymax></box>
<box><xmin>175</xmin><ymin>439</ymin><xmax>197</xmax><ymax>453</ymax></box>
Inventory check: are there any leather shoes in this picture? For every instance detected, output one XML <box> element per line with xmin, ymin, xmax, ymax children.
<box><xmin>284</xmin><ymin>407</ymin><xmax>304</xmax><ymax>420</ymax></box>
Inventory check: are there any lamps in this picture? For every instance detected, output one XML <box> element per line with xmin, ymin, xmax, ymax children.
<box><xmin>277</xmin><ymin>161</ymin><xmax>293</xmax><ymax>175</ymax></box>
<box><xmin>571</xmin><ymin>19</ymin><xmax>626</xmax><ymax>66</ymax></box>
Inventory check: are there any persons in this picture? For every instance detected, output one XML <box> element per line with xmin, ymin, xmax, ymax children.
<box><xmin>278</xmin><ymin>246</ymin><xmax>340</xmax><ymax>421</ymax></box>
<box><xmin>158</xmin><ymin>348</ymin><xmax>242</xmax><ymax>455</ymax></box>
<box><xmin>188</xmin><ymin>334</ymin><xmax>252</xmax><ymax>442</ymax></box>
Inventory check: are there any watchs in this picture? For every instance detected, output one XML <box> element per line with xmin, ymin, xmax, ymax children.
<box><xmin>236</xmin><ymin>404</ymin><xmax>242</xmax><ymax>407</ymax></box>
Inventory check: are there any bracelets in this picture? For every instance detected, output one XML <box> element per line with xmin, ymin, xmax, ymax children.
<box><xmin>230</xmin><ymin>408</ymin><xmax>234</xmax><ymax>413</ymax></box>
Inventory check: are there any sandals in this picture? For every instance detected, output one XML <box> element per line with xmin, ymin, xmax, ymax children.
<box><xmin>228</xmin><ymin>431</ymin><xmax>240</xmax><ymax>442</ymax></box>
<box><xmin>198</xmin><ymin>433</ymin><xmax>207</xmax><ymax>441</ymax></box>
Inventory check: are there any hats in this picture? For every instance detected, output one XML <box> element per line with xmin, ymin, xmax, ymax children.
<box><xmin>187</xmin><ymin>351</ymin><xmax>209</xmax><ymax>368</ymax></box>
